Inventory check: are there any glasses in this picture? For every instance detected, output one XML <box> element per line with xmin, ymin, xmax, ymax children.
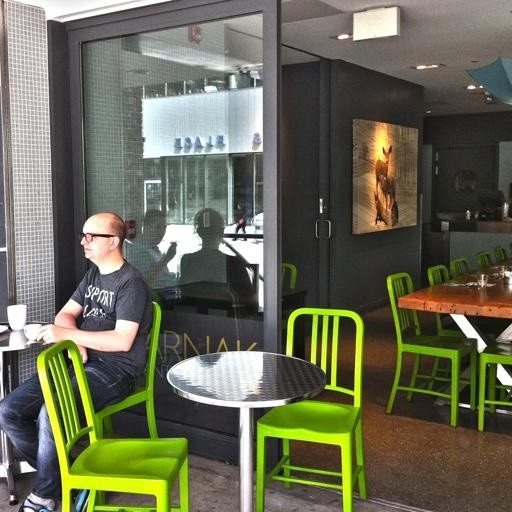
<box><xmin>78</xmin><ymin>232</ymin><xmax>116</xmax><ymax>242</ymax></box>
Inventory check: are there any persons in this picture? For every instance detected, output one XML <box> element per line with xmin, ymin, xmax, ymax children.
<box><xmin>180</xmin><ymin>207</ymin><xmax>258</xmax><ymax>304</ymax></box>
<box><xmin>231</xmin><ymin>203</ymin><xmax>247</xmax><ymax>241</ymax></box>
<box><xmin>0</xmin><ymin>211</ymin><xmax>155</xmax><ymax>512</ymax></box>
<box><xmin>127</xmin><ymin>209</ymin><xmax>180</xmax><ymax>301</ymax></box>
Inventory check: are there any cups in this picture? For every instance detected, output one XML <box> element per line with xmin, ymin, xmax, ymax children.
<box><xmin>8</xmin><ymin>331</ymin><xmax>27</xmax><ymax>350</ymax></box>
<box><xmin>480</xmin><ymin>289</ymin><xmax>488</xmax><ymax>303</ymax></box>
<box><xmin>480</xmin><ymin>273</ymin><xmax>488</xmax><ymax>288</ymax></box>
<box><xmin>499</xmin><ymin>271</ymin><xmax>504</xmax><ymax>280</ymax></box>
<box><xmin>7</xmin><ymin>305</ymin><xmax>42</xmax><ymax>340</ymax></box>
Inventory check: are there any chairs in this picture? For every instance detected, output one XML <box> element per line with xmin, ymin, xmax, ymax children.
<box><xmin>386</xmin><ymin>241</ymin><xmax>512</xmax><ymax>432</ymax></box>
<box><xmin>255</xmin><ymin>305</ymin><xmax>366</xmax><ymax>512</ymax></box>
<box><xmin>278</xmin><ymin>262</ymin><xmax>298</xmax><ymax>289</ymax></box>
<box><xmin>36</xmin><ymin>340</ymin><xmax>190</xmax><ymax>511</ymax></box>
<box><xmin>37</xmin><ymin>301</ymin><xmax>161</xmax><ymax>512</ymax></box>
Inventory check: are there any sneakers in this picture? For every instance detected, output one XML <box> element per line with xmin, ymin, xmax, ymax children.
<box><xmin>22</xmin><ymin>493</ymin><xmax>57</xmax><ymax>512</ymax></box>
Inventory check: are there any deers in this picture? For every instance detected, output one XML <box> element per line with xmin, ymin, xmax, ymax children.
<box><xmin>379</xmin><ymin>175</ymin><xmax>397</xmax><ymax>210</ymax></box>
<box><xmin>373</xmin><ymin>145</ymin><xmax>393</xmax><ymax>192</ymax></box>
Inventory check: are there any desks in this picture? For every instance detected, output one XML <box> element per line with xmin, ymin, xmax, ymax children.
<box><xmin>167</xmin><ymin>349</ymin><xmax>328</xmax><ymax>511</ymax></box>
<box><xmin>152</xmin><ymin>280</ymin><xmax>308</xmax><ymax>361</ymax></box>
